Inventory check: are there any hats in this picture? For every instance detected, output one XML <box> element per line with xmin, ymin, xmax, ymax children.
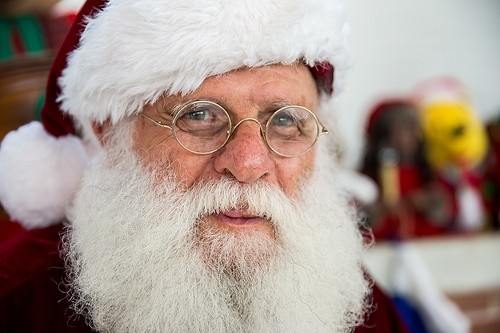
<box><xmin>363</xmin><ymin>101</ymin><xmax>416</xmax><ymax>143</ymax></box>
<box><xmin>0</xmin><ymin>0</ymin><xmax>359</xmax><ymax>236</ymax></box>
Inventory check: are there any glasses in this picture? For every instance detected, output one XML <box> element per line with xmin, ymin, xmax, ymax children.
<box><xmin>134</xmin><ymin>91</ymin><xmax>328</xmax><ymax>157</ymax></box>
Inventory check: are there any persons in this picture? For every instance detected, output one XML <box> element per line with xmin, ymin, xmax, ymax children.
<box><xmin>1</xmin><ymin>0</ymin><xmax>407</xmax><ymax>333</ymax></box>
